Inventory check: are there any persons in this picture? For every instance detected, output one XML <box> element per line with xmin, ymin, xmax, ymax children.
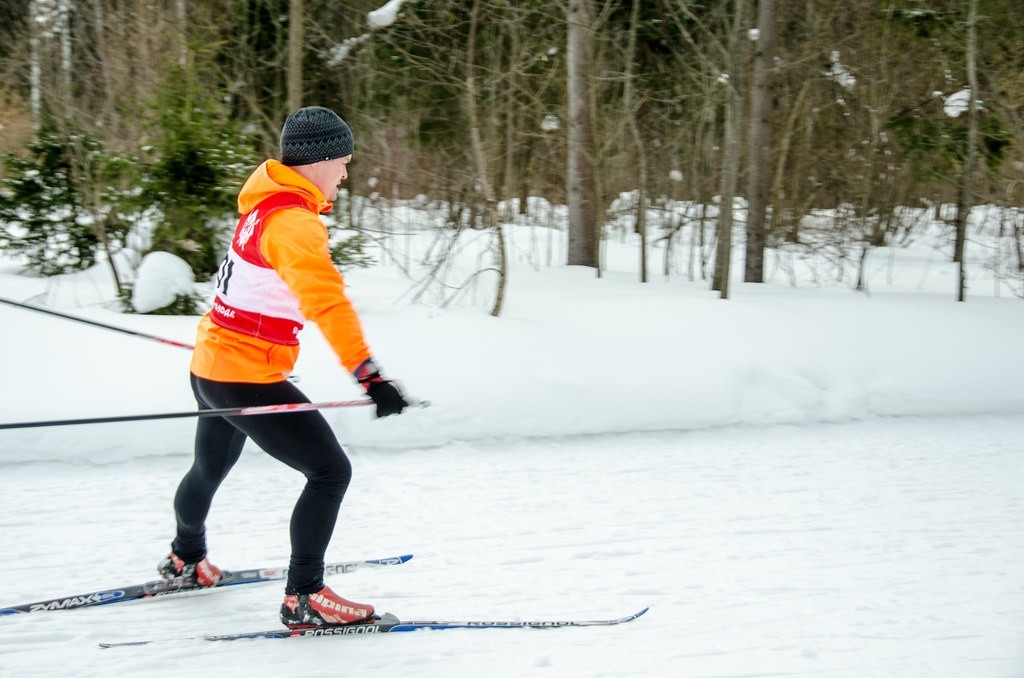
<box><xmin>159</xmin><ymin>106</ymin><xmax>404</xmax><ymax>627</ymax></box>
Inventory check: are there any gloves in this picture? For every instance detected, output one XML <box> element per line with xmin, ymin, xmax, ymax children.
<box><xmin>366</xmin><ymin>378</ymin><xmax>409</xmax><ymax>417</ymax></box>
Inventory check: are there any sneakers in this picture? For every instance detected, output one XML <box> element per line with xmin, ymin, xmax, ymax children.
<box><xmin>280</xmin><ymin>584</ymin><xmax>374</xmax><ymax>629</ymax></box>
<box><xmin>158</xmin><ymin>552</ymin><xmax>232</xmax><ymax>586</ymax></box>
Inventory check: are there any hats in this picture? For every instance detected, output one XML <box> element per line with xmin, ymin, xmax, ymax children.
<box><xmin>280</xmin><ymin>107</ymin><xmax>355</xmax><ymax>166</ymax></box>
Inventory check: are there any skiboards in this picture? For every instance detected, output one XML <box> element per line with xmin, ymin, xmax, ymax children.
<box><xmin>0</xmin><ymin>551</ymin><xmax>654</xmax><ymax>652</ymax></box>
